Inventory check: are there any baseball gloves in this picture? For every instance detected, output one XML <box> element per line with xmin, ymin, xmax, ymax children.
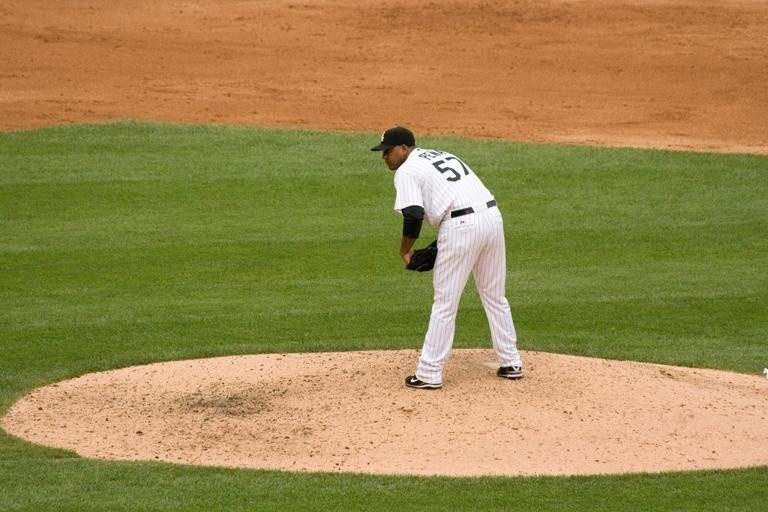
<box><xmin>406</xmin><ymin>240</ymin><xmax>436</xmax><ymax>270</ymax></box>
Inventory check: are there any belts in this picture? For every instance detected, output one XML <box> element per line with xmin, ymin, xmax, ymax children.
<box><xmin>451</xmin><ymin>201</ymin><xmax>496</xmax><ymax>217</ymax></box>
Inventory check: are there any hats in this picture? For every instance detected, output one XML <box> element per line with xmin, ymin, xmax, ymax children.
<box><xmin>370</xmin><ymin>128</ymin><xmax>415</xmax><ymax>151</ymax></box>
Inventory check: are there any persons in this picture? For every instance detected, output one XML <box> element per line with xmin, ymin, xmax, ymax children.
<box><xmin>371</xmin><ymin>125</ymin><xmax>523</xmax><ymax>389</ymax></box>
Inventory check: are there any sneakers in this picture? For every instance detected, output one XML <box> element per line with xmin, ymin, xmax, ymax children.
<box><xmin>497</xmin><ymin>367</ymin><xmax>522</xmax><ymax>378</ymax></box>
<box><xmin>406</xmin><ymin>376</ymin><xmax>441</xmax><ymax>388</ymax></box>
